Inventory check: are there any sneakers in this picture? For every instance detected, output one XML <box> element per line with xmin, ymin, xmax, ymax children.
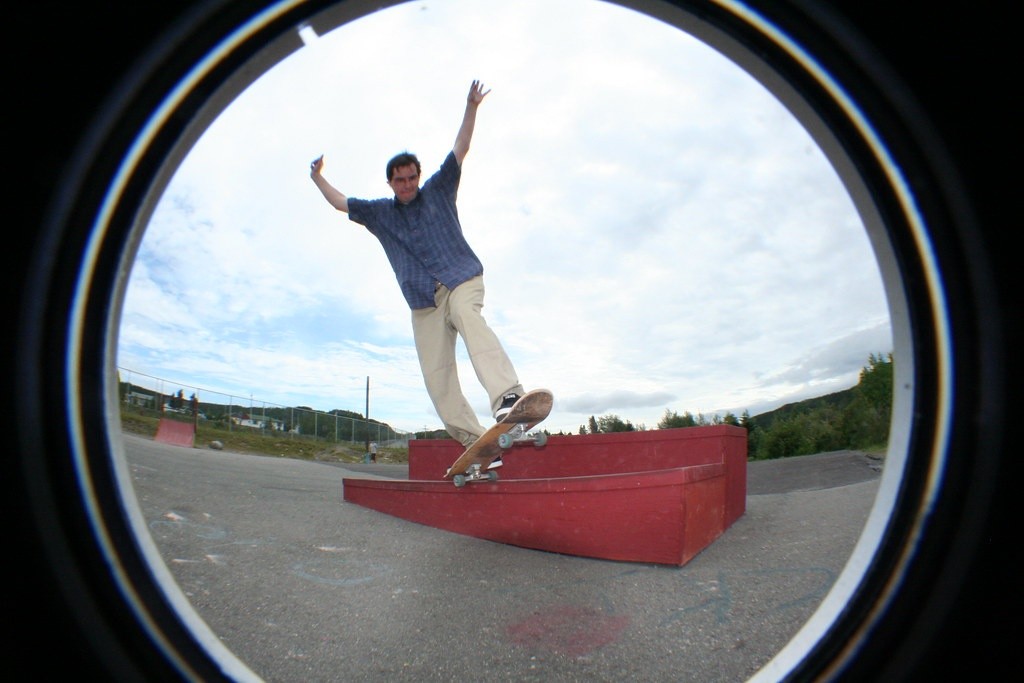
<box><xmin>447</xmin><ymin>456</ymin><xmax>503</xmax><ymax>474</ymax></box>
<box><xmin>496</xmin><ymin>393</ymin><xmax>521</xmax><ymax>423</ymax></box>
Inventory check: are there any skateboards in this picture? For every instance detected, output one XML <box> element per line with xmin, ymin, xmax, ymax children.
<box><xmin>444</xmin><ymin>389</ymin><xmax>554</xmax><ymax>487</ymax></box>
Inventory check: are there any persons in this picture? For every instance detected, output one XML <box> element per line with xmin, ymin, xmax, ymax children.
<box><xmin>369</xmin><ymin>440</ymin><xmax>379</xmax><ymax>463</ymax></box>
<box><xmin>308</xmin><ymin>75</ymin><xmax>527</xmax><ymax>451</ymax></box>
